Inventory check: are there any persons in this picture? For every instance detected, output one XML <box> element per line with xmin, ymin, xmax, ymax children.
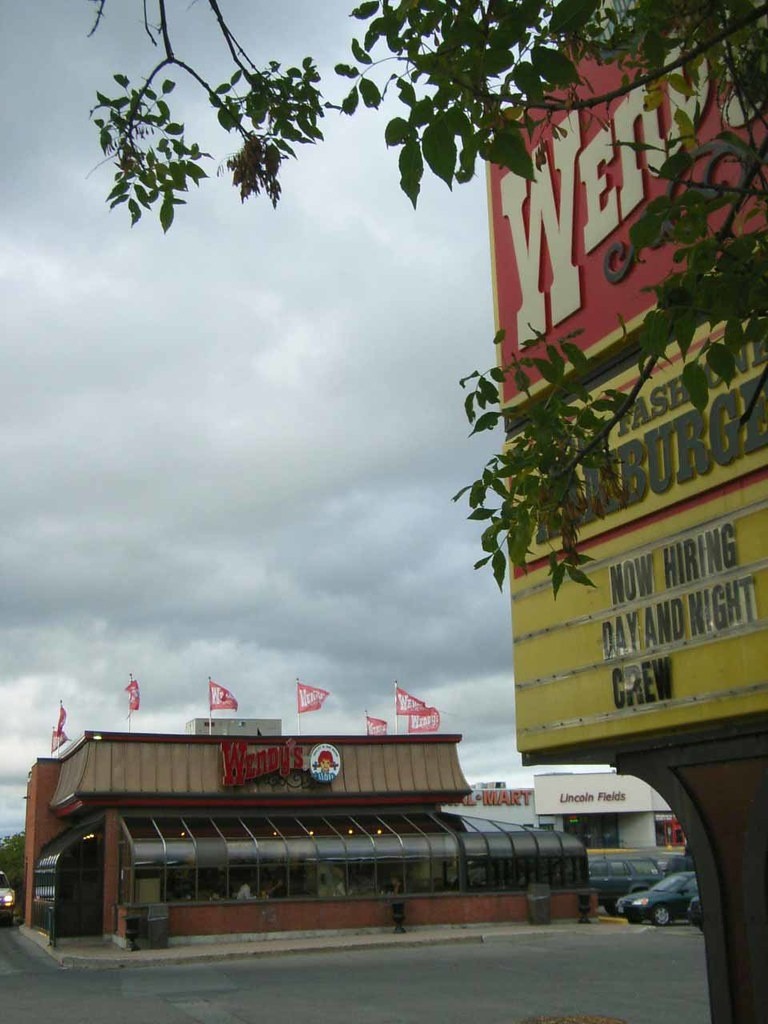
<box><xmin>333</xmin><ymin>876</ymin><xmax>353</xmax><ymax>896</ymax></box>
<box><xmin>385</xmin><ymin>871</ymin><xmax>404</xmax><ymax>895</ymax></box>
<box><xmin>237</xmin><ymin>880</ymin><xmax>257</xmax><ymax>900</ymax></box>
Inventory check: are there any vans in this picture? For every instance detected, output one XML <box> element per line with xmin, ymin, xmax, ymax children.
<box><xmin>589</xmin><ymin>857</ymin><xmax>664</xmax><ymax>916</ymax></box>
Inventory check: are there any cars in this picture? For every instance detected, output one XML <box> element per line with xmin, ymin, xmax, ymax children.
<box><xmin>616</xmin><ymin>871</ymin><xmax>699</xmax><ymax>926</ymax></box>
<box><xmin>0</xmin><ymin>871</ymin><xmax>16</xmax><ymax>926</ymax></box>
<box><xmin>687</xmin><ymin>896</ymin><xmax>705</xmax><ymax>932</ymax></box>
<box><xmin>33</xmin><ymin>867</ymin><xmax>56</xmax><ymax>916</ymax></box>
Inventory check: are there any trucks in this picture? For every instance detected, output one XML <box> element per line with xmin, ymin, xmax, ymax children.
<box><xmin>656</xmin><ymin>817</ymin><xmax>686</xmax><ymax>846</ymax></box>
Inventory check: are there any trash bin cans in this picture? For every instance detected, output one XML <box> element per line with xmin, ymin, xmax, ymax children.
<box><xmin>142</xmin><ymin>904</ymin><xmax>170</xmax><ymax>950</ymax></box>
<box><xmin>527</xmin><ymin>882</ymin><xmax>551</xmax><ymax>926</ymax></box>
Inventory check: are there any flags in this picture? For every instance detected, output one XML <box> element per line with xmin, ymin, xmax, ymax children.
<box><xmin>297</xmin><ymin>682</ymin><xmax>330</xmax><ymax>713</ymax></box>
<box><xmin>125</xmin><ymin>680</ymin><xmax>138</xmax><ymax>710</ymax></box>
<box><xmin>51</xmin><ymin>707</ymin><xmax>71</xmax><ymax>753</ymax></box>
<box><xmin>366</xmin><ymin>716</ymin><xmax>387</xmax><ymax>735</ymax></box>
<box><xmin>396</xmin><ymin>688</ymin><xmax>440</xmax><ymax>734</ymax></box>
<box><xmin>208</xmin><ymin>681</ymin><xmax>238</xmax><ymax>712</ymax></box>
